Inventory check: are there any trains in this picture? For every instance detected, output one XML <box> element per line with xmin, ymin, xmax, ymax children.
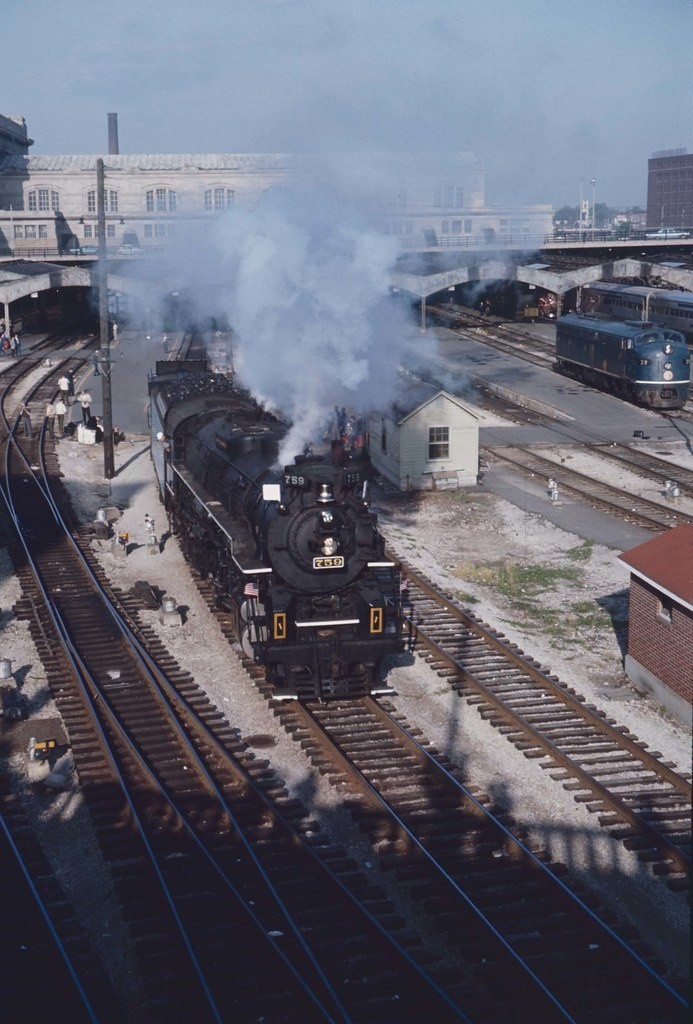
<box><xmin>553</xmin><ymin>312</ymin><xmax>690</xmax><ymax>410</ymax></box>
<box><xmin>564</xmin><ymin>280</ymin><xmax>693</xmax><ymax>343</ymax></box>
<box><xmin>142</xmin><ymin>297</ymin><xmax>421</xmax><ymax>702</ymax></box>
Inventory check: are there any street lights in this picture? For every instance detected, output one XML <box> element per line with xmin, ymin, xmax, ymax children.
<box><xmin>591</xmin><ymin>178</ymin><xmax>596</xmax><ymax>243</ymax></box>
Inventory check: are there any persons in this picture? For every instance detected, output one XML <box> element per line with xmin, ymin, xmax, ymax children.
<box><xmin>44</xmin><ymin>401</ymin><xmax>56</xmax><ymax>438</ymax></box>
<box><xmin>1</xmin><ymin>319</ymin><xmax>21</xmax><ymax>358</ymax></box>
<box><xmin>19</xmin><ymin>403</ymin><xmax>33</xmax><ymax>439</ymax></box>
<box><xmin>54</xmin><ymin>399</ymin><xmax>67</xmax><ymax>435</ymax></box>
<box><xmin>77</xmin><ymin>390</ymin><xmax>92</xmax><ymax>427</ymax></box>
<box><xmin>92</xmin><ymin>350</ymin><xmax>101</xmax><ymax>376</ymax></box>
<box><xmin>86</xmin><ymin>416</ymin><xmax>104</xmax><ymax>443</ymax></box>
<box><xmin>161</xmin><ymin>333</ymin><xmax>168</xmax><ymax>354</ymax></box>
<box><xmin>113</xmin><ymin>321</ymin><xmax>118</xmax><ymax>342</ymax></box>
<box><xmin>58</xmin><ymin>368</ymin><xmax>75</xmax><ymax>407</ymax></box>
<box><xmin>156</xmin><ymin>431</ymin><xmax>183</xmax><ymax>461</ymax></box>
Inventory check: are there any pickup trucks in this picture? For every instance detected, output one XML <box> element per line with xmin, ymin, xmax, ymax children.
<box><xmin>646</xmin><ymin>228</ymin><xmax>689</xmax><ymax>240</ymax></box>
